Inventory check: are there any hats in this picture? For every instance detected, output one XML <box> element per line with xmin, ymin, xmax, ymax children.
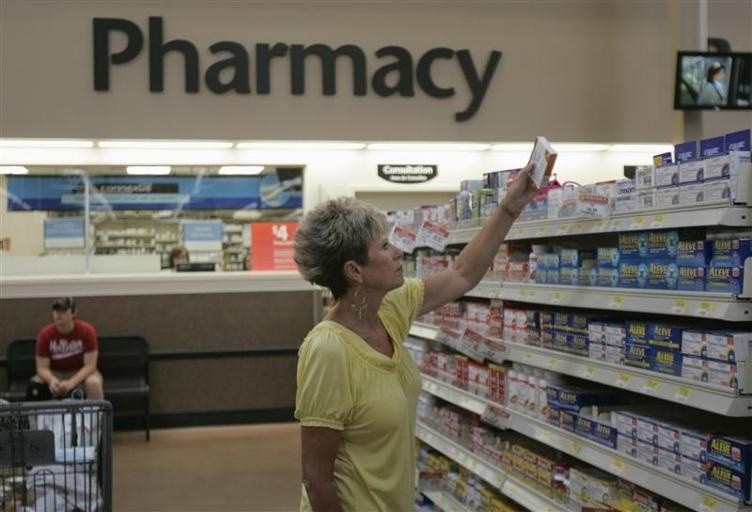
<box><xmin>51</xmin><ymin>299</ymin><xmax>75</xmax><ymax>311</ymax></box>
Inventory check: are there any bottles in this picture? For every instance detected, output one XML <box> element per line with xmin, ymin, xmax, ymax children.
<box><xmin>527</xmin><ymin>244</ymin><xmax>550</xmax><ymax>283</ymax></box>
<box><xmin>504</xmin><ymin>362</ymin><xmax>562</xmax><ymax>423</ymax></box>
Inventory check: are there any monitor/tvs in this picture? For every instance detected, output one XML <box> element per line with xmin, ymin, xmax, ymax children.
<box><xmin>674</xmin><ymin>50</ymin><xmax>752</xmax><ymax>110</ymax></box>
<box><xmin>177</xmin><ymin>262</ymin><xmax>215</xmax><ymax>272</ymax></box>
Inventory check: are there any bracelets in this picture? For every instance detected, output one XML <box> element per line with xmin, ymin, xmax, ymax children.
<box><xmin>497</xmin><ymin>199</ymin><xmax>518</xmax><ymax>220</ymax></box>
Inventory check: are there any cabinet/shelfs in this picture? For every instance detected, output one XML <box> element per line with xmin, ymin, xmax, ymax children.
<box><xmin>322</xmin><ymin>163</ymin><xmax>752</xmax><ymax>512</ymax></box>
<box><xmin>95</xmin><ymin>218</ymin><xmax>251</xmax><ymax>269</ymax></box>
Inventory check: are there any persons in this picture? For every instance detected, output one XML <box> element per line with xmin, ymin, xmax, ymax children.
<box><xmin>697</xmin><ymin>61</ymin><xmax>726</xmax><ymax>105</ymax></box>
<box><xmin>26</xmin><ymin>293</ymin><xmax>104</xmax><ymax>430</ymax></box>
<box><xmin>293</xmin><ymin>161</ymin><xmax>533</xmax><ymax>512</ymax></box>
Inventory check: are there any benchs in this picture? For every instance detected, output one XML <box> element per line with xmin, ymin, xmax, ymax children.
<box><xmin>8</xmin><ymin>337</ymin><xmax>151</xmax><ymax>441</ymax></box>
